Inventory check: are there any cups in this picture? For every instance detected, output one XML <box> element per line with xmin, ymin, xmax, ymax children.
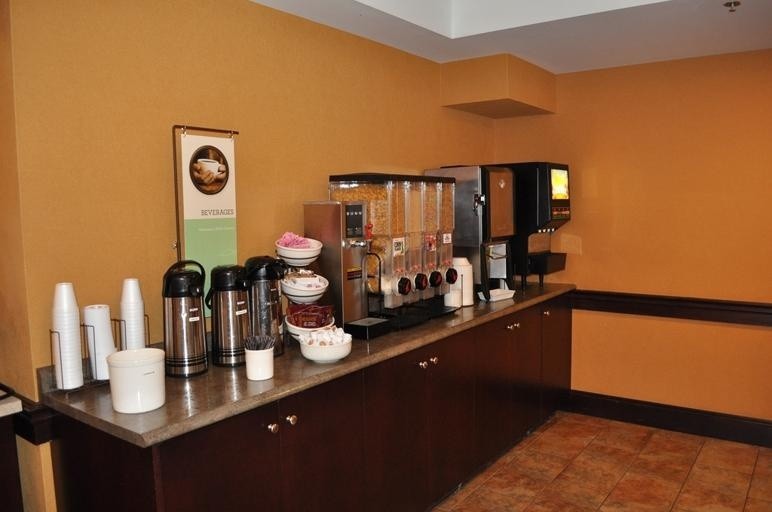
<box><xmin>196</xmin><ymin>158</ymin><xmax>223</xmax><ymax>181</ymax></box>
<box><xmin>52</xmin><ymin>278</ymin><xmax>146</xmax><ymax>391</ymax></box>
<box><xmin>443</xmin><ymin>274</ymin><xmax>463</xmax><ymax>309</ymax></box>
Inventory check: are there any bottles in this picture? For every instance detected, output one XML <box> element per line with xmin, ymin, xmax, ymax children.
<box><xmin>105</xmin><ymin>348</ymin><xmax>166</xmax><ymax>415</ymax></box>
<box><xmin>443</xmin><ymin>257</ymin><xmax>474</xmax><ymax>307</ymax></box>
<box><xmin>161</xmin><ymin>256</ymin><xmax>285</xmax><ymax>378</ymax></box>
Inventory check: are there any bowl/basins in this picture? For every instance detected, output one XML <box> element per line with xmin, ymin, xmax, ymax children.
<box><xmin>274</xmin><ymin>237</ymin><xmax>352</xmax><ymax>364</ymax></box>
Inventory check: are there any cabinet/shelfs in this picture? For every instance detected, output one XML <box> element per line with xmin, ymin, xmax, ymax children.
<box><xmin>475</xmin><ymin>301</ymin><xmax>543</xmax><ymax>468</ymax></box>
<box><xmin>46</xmin><ymin>368</ymin><xmax>363</xmax><ymax>511</ymax></box>
<box><xmin>363</xmin><ymin>325</ymin><xmax>475</xmax><ymax>512</ymax></box>
<box><xmin>543</xmin><ymin>290</ymin><xmax>572</xmax><ymax>420</ymax></box>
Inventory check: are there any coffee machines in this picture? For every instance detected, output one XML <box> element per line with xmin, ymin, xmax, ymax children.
<box><xmin>302</xmin><ymin>200</ymin><xmax>389</xmax><ymax>340</ymax></box>
<box><xmin>422</xmin><ymin>162</ymin><xmax>518</xmax><ymax>301</ymax></box>
<box><xmin>513</xmin><ymin>162</ymin><xmax>570</xmax><ymax>291</ymax></box>
